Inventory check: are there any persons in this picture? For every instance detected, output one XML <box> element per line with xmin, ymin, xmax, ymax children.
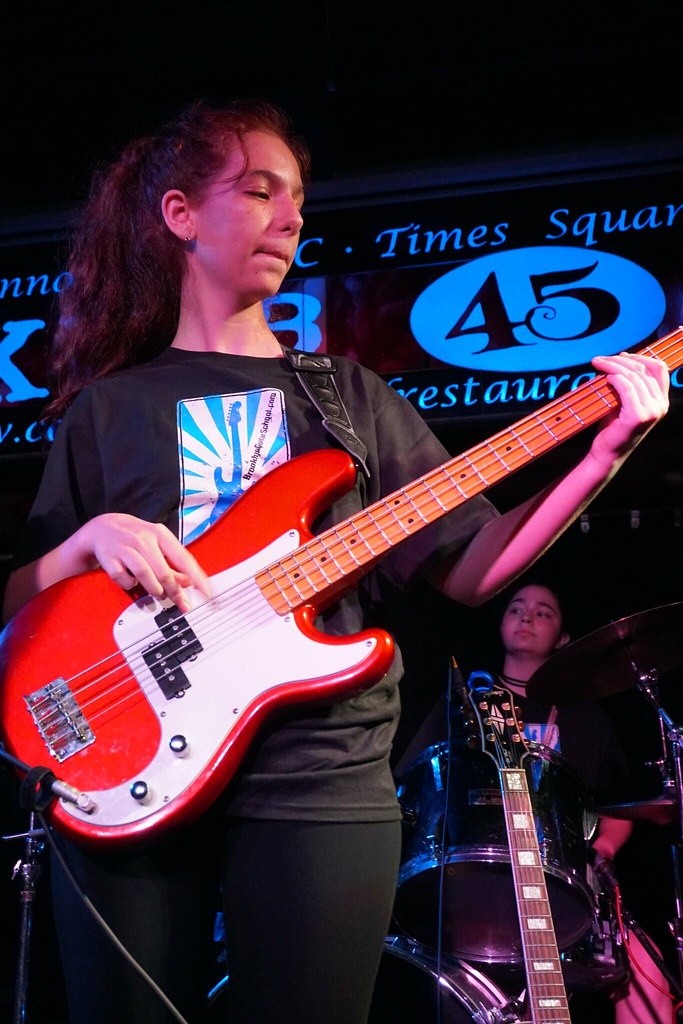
<box><xmin>0</xmin><ymin>92</ymin><xmax>671</xmax><ymax>1024</ymax></box>
<box><xmin>392</xmin><ymin>577</ymin><xmax>634</xmax><ymax>1024</ymax></box>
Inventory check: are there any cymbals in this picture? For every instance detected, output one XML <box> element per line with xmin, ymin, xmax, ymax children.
<box><xmin>524</xmin><ymin>600</ymin><xmax>683</xmax><ymax>704</ymax></box>
<box><xmin>593</xmin><ymin>795</ymin><xmax>682</xmax><ymax>828</ymax></box>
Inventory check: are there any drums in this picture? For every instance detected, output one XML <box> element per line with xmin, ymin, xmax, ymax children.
<box><xmin>364</xmin><ymin>740</ymin><xmax>674</xmax><ymax>1024</ymax></box>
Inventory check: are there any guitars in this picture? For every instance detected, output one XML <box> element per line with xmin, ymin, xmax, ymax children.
<box><xmin>456</xmin><ymin>667</ymin><xmax>574</xmax><ymax>1024</ymax></box>
<box><xmin>0</xmin><ymin>316</ymin><xmax>683</xmax><ymax>845</ymax></box>
